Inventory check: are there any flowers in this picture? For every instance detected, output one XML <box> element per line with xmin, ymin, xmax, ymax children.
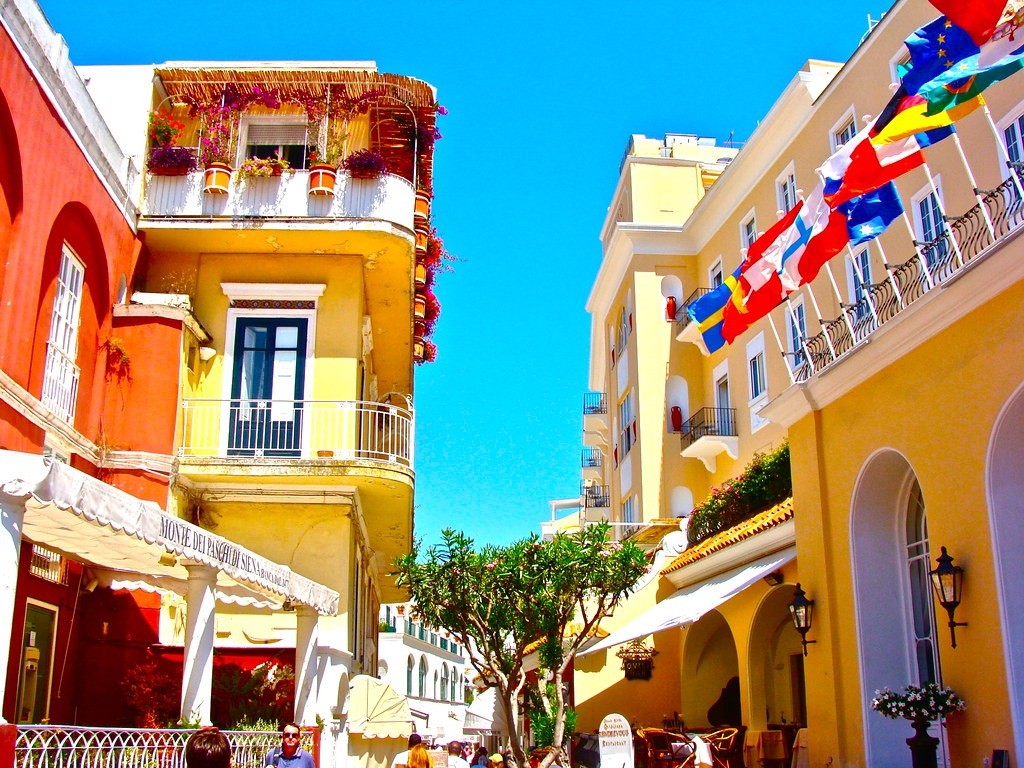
<box><xmin>425</xmin><ymin>228</ymin><xmax>467</xmax><ymax>364</ymax></box>
<box><xmin>871</xmin><ymin>684</ymin><xmax>967</xmax><ymax>728</ymax></box>
<box><xmin>149</xmin><ymin>83</ymin><xmax>450</xmax><ymax>188</ymax></box>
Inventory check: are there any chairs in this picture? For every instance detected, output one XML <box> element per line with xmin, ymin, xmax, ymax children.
<box><xmin>701</xmin><ymin>727</ymin><xmax>739</xmax><ymax>768</ymax></box>
<box><xmin>636</xmin><ymin>727</ymin><xmax>664</xmax><ymax>739</ymax></box>
<box><xmin>645</xmin><ymin>730</ymin><xmax>697</xmax><ymax>768</ymax></box>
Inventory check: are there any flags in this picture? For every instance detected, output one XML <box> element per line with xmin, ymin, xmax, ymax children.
<box><xmin>677</xmin><ymin>0</ymin><xmax>1024</xmax><ymax>357</ymax></box>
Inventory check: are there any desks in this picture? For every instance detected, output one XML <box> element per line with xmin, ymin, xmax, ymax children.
<box><xmin>670</xmin><ymin>742</ymin><xmax>714</xmax><ymax>768</ymax></box>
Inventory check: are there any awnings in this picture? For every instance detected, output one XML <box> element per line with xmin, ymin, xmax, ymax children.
<box><xmin>349</xmin><ymin>672</ymin><xmax>413</xmax><ymax>741</ymax></box>
<box><xmin>574</xmin><ymin>545</ymin><xmax>798</xmax><ymax>658</ymax></box>
<box><xmin>463</xmin><ymin>686</ymin><xmax>518</xmax><ymax>729</ymax></box>
<box><xmin>472</xmin><ymin>625</ymin><xmax>609</xmax><ymax>690</ymax></box>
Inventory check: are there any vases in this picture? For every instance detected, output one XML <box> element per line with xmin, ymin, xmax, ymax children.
<box><xmin>414</xmin><ymin>322</ymin><xmax>428</xmax><ymax>342</ymax></box>
<box><xmin>905</xmin><ymin>722</ymin><xmax>940</xmax><ymax>768</ymax></box>
<box><xmin>415</xmin><ymin>189</ymin><xmax>431</xmax><ymax>225</ymax></box>
<box><xmin>203</xmin><ymin>162</ymin><xmax>232</xmax><ymax>195</ymax></box>
<box><xmin>414</xmin><ymin>223</ymin><xmax>428</xmax><ymax>261</ymax></box>
<box><xmin>415</xmin><ymin>264</ymin><xmax>427</xmax><ymax>290</ymax></box>
<box><xmin>268</xmin><ymin>162</ymin><xmax>283</xmax><ymax>177</ymax></box>
<box><xmin>415</xmin><ymin>292</ymin><xmax>427</xmax><ymax>319</ymax></box>
<box><xmin>152</xmin><ymin>164</ymin><xmax>189</xmax><ymax>176</ymax></box>
<box><xmin>309</xmin><ymin>163</ymin><xmax>336</xmax><ymax>196</ymax></box>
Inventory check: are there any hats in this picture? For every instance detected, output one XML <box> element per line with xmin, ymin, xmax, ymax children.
<box><xmin>489</xmin><ymin>754</ymin><xmax>503</xmax><ymax>763</ymax></box>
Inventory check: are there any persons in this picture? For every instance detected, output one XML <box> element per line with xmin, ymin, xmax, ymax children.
<box><xmin>186</xmin><ymin>727</ymin><xmax>231</xmax><ymax>768</ymax></box>
<box><xmin>264</xmin><ymin>722</ymin><xmax>317</xmax><ymax>768</ymax></box>
<box><xmin>707</xmin><ymin>675</ymin><xmax>741</xmax><ymax>725</ymax></box>
<box><xmin>390</xmin><ymin>733</ymin><xmax>564</xmax><ymax>768</ymax></box>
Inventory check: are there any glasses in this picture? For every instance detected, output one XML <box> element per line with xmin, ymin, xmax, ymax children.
<box><xmin>283</xmin><ymin>733</ymin><xmax>299</xmax><ymax>738</ymax></box>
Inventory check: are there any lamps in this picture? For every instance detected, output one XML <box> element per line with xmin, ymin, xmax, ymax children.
<box><xmin>928</xmin><ymin>545</ymin><xmax>968</xmax><ymax>650</ymax></box>
<box><xmin>788</xmin><ymin>582</ymin><xmax>816</xmax><ymax>657</ymax></box>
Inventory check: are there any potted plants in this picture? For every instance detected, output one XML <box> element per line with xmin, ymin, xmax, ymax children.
<box><xmin>414</xmin><ymin>343</ymin><xmax>424</xmax><ymax>361</ymax></box>
